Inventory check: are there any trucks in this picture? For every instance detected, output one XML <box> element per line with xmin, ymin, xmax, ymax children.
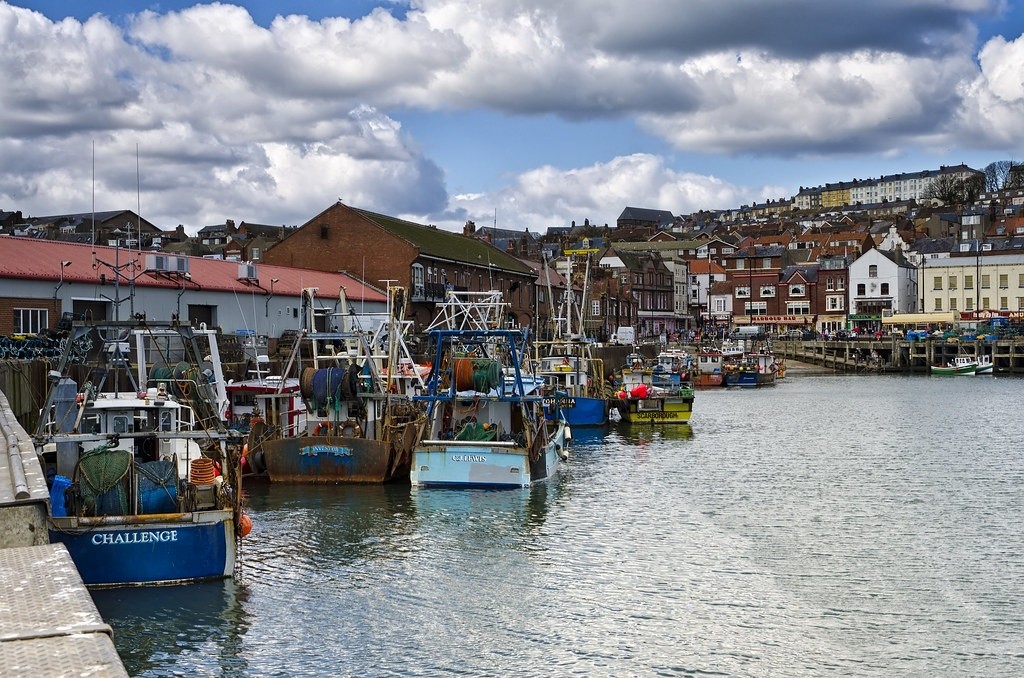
<box><xmin>616</xmin><ymin>327</ymin><xmax>635</xmax><ymax>345</ymax></box>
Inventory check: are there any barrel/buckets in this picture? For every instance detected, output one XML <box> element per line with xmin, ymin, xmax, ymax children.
<box><xmin>192</xmin><ymin>457</ymin><xmax>215</xmax><ymax>486</ymax></box>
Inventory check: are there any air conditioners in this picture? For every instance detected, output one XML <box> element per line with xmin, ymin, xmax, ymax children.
<box><xmin>236</xmin><ymin>265</ymin><xmax>257</xmax><ymax>280</ymax></box>
<box><xmin>167</xmin><ymin>256</ymin><xmax>189</xmax><ymax>273</ymax></box>
<box><xmin>145</xmin><ymin>254</ymin><xmax>166</xmax><ymax>272</ymax></box>
<box><xmin>203</xmin><ymin>254</ymin><xmax>223</xmax><ymax>260</ymax></box>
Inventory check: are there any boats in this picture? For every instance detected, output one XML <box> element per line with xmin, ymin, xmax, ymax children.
<box><xmin>931</xmin><ymin>355</ymin><xmax>994</xmax><ymax>376</ymax></box>
<box><xmin>613</xmin><ymin>320</ymin><xmax>787</xmax><ymax>385</ymax></box>
<box><xmin>31</xmin><ymin>139</ymin><xmax>248</xmax><ymax>592</ymax></box>
<box><xmin>410</xmin><ymin>251</ymin><xmax>695</xmax><ymax>487</ymax></box>
<box><xmin>225</xmin><ymin>258</ymin><xmax>431</xmax><ymax>483</ymax></box>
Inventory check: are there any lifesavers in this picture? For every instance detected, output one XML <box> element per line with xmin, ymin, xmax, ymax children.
<box><xmin>404</xmin><ymin>364</ymin><xmax>432</xmax><ymax>376</ymax></box>
<box><xmin>339</xmin><ymin>421</ymin><xmax>362</xmax><ymax>438</ymax></box>
<box><xmin>314</xmin><ymin>421</ymin><xmax>336</xmax><ymax>437</ymax></box>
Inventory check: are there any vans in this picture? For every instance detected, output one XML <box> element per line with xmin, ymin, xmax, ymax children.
<box><xmin>728</xmin><ymin>327</ymin><xmax>764</xmax><ymax>341</ymax></box>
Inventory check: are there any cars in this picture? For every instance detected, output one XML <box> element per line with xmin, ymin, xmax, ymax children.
<box><xmin>564</xmin><ymin>338</ymin><xmax>603</xmax><ymax>348</ymax></box>
<box><xmin>778</xmin><ymin>330</ymin><xmax>813</xmax><ymax>341</ymax></box>
<box><xmin>828</xmin><ymin>330</ymin><xmax>858</xmax><ymax>341</ymax></box>
